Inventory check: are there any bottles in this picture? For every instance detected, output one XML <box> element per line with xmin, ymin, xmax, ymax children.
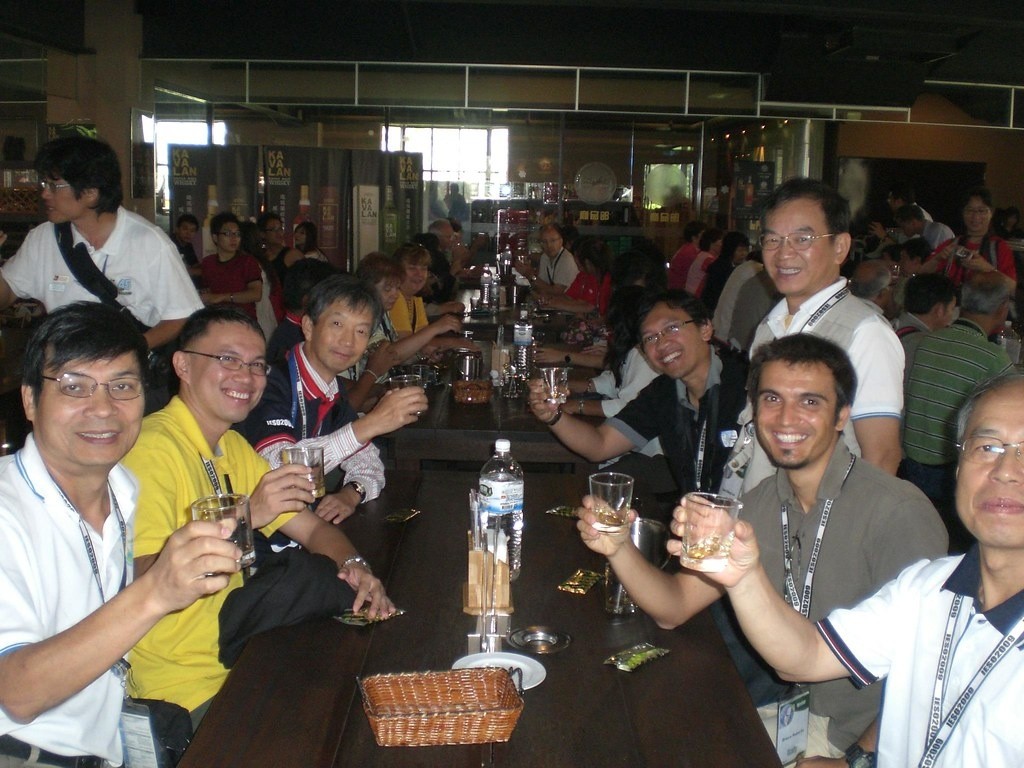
<box><xmin>480</xmin><ymin>267</ymin><xmax>492</xmax><ymax>303</ymax></box>
<box><xmin>454</xmin><ymin>350</ymin><xmax>479</xmax><ymax>381</ymax></box>
<box><xmin>293</xmin><ymin>185</ymin><xmax>314</xmax><ymax>249</ymax></box>
<box><xmin>202</xmin><ymin>185</ymin><xmax>222</xmax><ymax>258</ymax></box>
<box><xmin>380</xmin><ymin>186</ymin><xmax>399</xmax><ymax>253</ymax></box>
<box><xmin>744</xmin><ymin>176</ymin><xmax>753</xmax><ymax>207</ymax></box>
<box><xmin>228</xmin><ymin>183</ymin><xmax>248</xmax><ymax>222</ymax></box>
<box><xmin>514</xmin><ymin>310</ymin><xmax>532</xmax><ymax>379</ymax></box>
<box><xmin>997</xmin><ymin>320</ymin><xmax>1021</xmax><ymax>364</ymax></box>
<box><xmin>480</xmin><ymin>440</ymin><xmax>524</xmax><ymax>579</ymax></box>
<box><xmin>704</xmin><ymin>187</ymin><xmax>717</xmax><ymax>209</ymax></box>
<box><xmin>317</xmin><ymin>187</ymin><xmax>340</xmax><ymax>250</ymax></box>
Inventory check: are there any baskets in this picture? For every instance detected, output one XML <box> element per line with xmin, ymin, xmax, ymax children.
<box><xmin>357</xmin><ymin>667</ymin><xmax>525</xmax><ymax>745</ymax></box>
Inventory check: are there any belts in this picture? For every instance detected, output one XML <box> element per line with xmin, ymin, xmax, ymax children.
<box><xmin>0</xmin><ymin>735</ymin><xmax>101</xmax><ymax>767</ymax></box>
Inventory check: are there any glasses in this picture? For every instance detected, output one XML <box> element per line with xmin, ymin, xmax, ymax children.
<box><xmin>398</xmin><ymin>243</ymin><xmax>424</xmax><ymax>260</ymax></box>
<box><xmin>219</xmin><ymin>232</ymin><xmax>241</xmax><ymax>238</ymax></box>
<box><xmin>266</xmin><ymin>227</ymin><xmax>283</xmax><ymax>233</ymax></box>
<box><xmin>41</xmin><ymin>181</ymin><xmax>71</xmax><ymax>192</ymax></box>
<box><xmin>759</xmin><ymin>231</ymin><xmax>835</xmax><ymax>250</ymax></box>
<box><xmin>955</xmin><ymin>436</ymin><xmax>1024</xmax><ymax>464</ymax></box>
<box><xmin>182</xmin><ymin>350</ymin><xmax>271</xmax><ymax>376</ymax></box>
<box><xmin>43</xmin><ymin>372</ymin><xmax>146</xmax><ymax>401</ymax></box>
<box><xmin>641</xmin><ymin>318</ymin><xmax>696</xmax><ymax>344</ymax></box>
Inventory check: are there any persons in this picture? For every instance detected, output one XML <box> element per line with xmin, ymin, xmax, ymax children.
<box><xmin>119</xmin><ymin>300</ymin><xmax>395</xmax><ymax>732</ymax></box>
<box><xmin>167</xmin><ymin>211</ymin><xmax>329</xmax><ymax>342</ymax></box>
<box><xmin>665</xmin><ymin>374</ymin><xmax>1024</xmax><ymax>768</ymax></box>
<box><xmin>0</xmin><ymin>300</ymin><xmax>242</xmax><ymax>768</ymax></box>
<box><xmin>0</xmin><ymin>133</ymin><xmax>205</xmax><ymax>389</ymax></box>
<box><xmin>919</xmin><ymin>185</ymin><xmax>1016</xmax><ymax>289</ymax></box>
<box><xmin>530</xmin><ymin>169</ymin><xmax>1024</xmax><ymax>532</ymax></box>
<box><xmin>854</xmin><ymin>186</ymin><xmax>1024</xmax><ymax>555</ymax></box>
<box><xmin>235</xmin><ymin>183</ymin><xmax>485</xmax><ymax>527</ymax></box>
<box><xmin>574</xmin><ymin>332</ymin><xmax>948</xmax><ymax>768</ymax></box>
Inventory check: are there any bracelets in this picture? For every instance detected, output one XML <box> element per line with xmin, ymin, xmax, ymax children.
<box><xmin>584</xmin><ymin>375</ymin><xmax>592</xmax><ymax>395</ymax></box>
<box><xmin>230</xmin><ymin>291</ymin><xmax>235</xmax><ymax>305</ymax></box>
<box><xmin>577</xmin><ymin>397</ymin><xmax>585</xmax><ymax>416</ymax></box>
<box><xmin>545</xmin><ymin>408</ymin><xmax>563</xmax><ymax>427</ymax></box>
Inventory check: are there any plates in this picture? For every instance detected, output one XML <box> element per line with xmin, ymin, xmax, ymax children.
<box><xmin>452</xmin><ymin>652</ymin><xmax>546</xmax><ymax>691</ymax></box>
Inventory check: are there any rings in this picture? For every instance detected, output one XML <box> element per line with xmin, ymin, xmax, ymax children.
<box><xmin>526</xmin><ymin>400</ymin><xmax>532</xmax><ymax>405</ymax></box>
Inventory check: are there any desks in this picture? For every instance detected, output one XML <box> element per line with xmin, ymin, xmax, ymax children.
<box><xmin>378</xmin><ymin>261</ymin><xmax>645</xmax><ymax>474</ymax></box>
<box><xmin>176</xmin><ymin>468</ymin><xmax>788</xmax><ymax>768</ymax></box>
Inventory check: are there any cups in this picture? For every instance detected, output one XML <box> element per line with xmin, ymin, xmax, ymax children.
<box><xmin>539</xmin><ymin>368</ymin><xmax>567</xmax><ymax>403</ymax></box>
<box><xmin>282</xmin><ymin>447</ymin><xmax>325</xmax><ymax>499</ymax></box>
<box><xmin>518</xmin><ymin>256</ymin><xmax>527</xmax><ymax>263</ymax></box>
<box><xmin>458</xmin><ymin>331</ymin><xmax>473</xmax><ymax>352</ymax></box>
<box><xmin>412</xmin><ymin>364</ymin><xmax>429</xmax><ymax>388</ymax></box>
<box><xmin>680</xmin><ymin>493</ymin><xmax>744</xmax><ymax>573</ymax></box>
<box><xmin>470</xmin><ymin>297</ymin><xmax>481</xmax><ymax>311</ymax></box>
<box><xmin>606</xmin><ymin>517</ymin><xmax>671</xmax><ymax>618</ymax></box>
<box><xmin>390</xmin><ymin>375</ymin><xmax>421</xmax><ymax>416</ymax></box>
<box><xmin>589</xmin><ymin>471</ymin><xmax>634</xmax><ymax>532</ymax></box>
<box><xmin>191</xmin><ymin>494</ymin><xmax>255</xmax><ymax>567</ymax></box>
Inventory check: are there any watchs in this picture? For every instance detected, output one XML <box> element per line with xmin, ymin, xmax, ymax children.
<box><xmin>564</xmin><ymin>352</ymin><xmax>571</xmax><ymax>364</ymax></box>
<box><xmin>337</xmin><ymin>554</ymin><xmax>374</xmax><ymax>575</ymax></box>
<box><xmin>344</xmin><ymin>480</ymin><xmax>366</xmax><ymax>502</ymax></box>
<box><xmin>843</xmin><ymin>742</ymin><xmax>875</xmax><ymax>768</ymax></box>
